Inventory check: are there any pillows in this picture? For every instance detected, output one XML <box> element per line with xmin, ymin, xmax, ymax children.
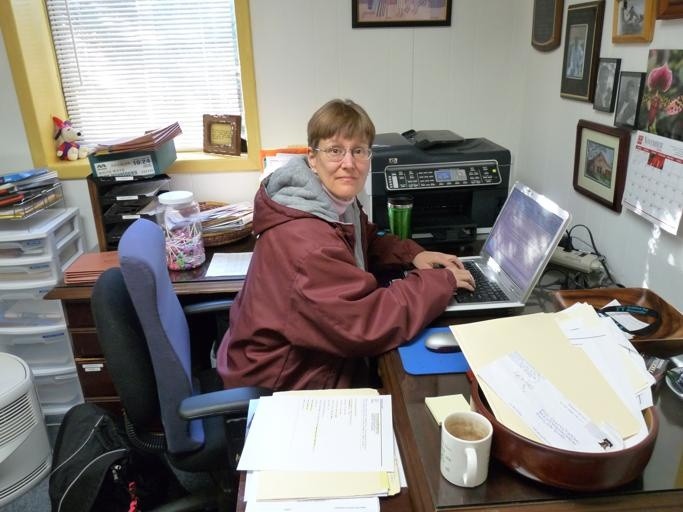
<box><xmin>117</xmin><ymin>219</ymin><xmax>206</xmax><ymax>453</ymax></box>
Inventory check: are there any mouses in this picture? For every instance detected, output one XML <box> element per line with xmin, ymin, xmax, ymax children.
<box><xmin>426</xmin><ymin>333</ymin><xmax>460</xmax><ymax>354</ymax></box>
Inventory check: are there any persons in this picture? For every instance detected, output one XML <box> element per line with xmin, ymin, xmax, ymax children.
<box><xmin>213</xmin><ymin>94</ymin><xmax>476</xmax><ymax>392</ymax></box>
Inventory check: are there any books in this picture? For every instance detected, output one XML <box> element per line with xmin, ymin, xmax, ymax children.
<box><xmin>0</xmin><ymin>167</ymin><xmax>59</xmax><ymax>221</ymax></box>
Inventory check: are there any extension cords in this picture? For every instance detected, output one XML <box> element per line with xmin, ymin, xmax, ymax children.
<box><xmin>551</xmin><ymin>246</ymin><xmax>600</xmax><ymax>274</ymax></box>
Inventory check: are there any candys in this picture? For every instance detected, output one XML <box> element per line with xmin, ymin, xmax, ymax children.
<box><xmin>159</xmin><ymin>222</ymin><xmax>204</xmax><ymax>270</ymax></box>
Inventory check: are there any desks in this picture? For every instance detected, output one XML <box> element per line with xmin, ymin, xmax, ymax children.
<box><xmin>42</xmin><ymin>234</ymin><xmax>683</xmax><ymax>511</ymax></box>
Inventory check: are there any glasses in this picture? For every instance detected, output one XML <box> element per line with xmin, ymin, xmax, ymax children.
<box><xmin>315</xmin><ymin>143</ymin><xmax>374</xmax><ymax>160</ymax></box>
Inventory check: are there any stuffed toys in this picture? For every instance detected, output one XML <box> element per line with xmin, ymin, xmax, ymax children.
<box><xmin>51</xmin><ymin>116</ymin><xmax>89</xmax><ymax>161</ymax></box>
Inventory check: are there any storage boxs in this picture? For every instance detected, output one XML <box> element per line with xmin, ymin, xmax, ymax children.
<box><xmin>87</xmin><ymin>138</ymin><xmax>178</xmax><ymax>179</ymax></box>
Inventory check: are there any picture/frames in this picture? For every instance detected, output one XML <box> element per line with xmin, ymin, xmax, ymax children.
<box><xmin>352</xmin><ymin>0</ymin><xmax>450</xmax><ymax>27</ymax></box>
<box><xmin>531</xmin><ymin>0</ymin><xmax>683</xmax><ymax>214</ymax></box>
<box><xmin>203</xmin><ymin>113</ymin><xmax>241</xmax><ymax>156</ymax></box>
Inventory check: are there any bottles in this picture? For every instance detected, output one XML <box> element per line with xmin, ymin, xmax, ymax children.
<box><xmin>154</xmin><ymin>189</ymin><xmax>207</xmax><ymax>270</ymax></box>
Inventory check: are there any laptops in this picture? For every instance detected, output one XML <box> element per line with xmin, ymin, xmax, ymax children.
<box><xmin>404</xmin><ymin>181</ymin><xmax>571</xmax><ymax>313</ymax></box>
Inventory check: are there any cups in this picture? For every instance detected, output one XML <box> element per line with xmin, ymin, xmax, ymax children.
<box><xmin>440</xmin><ymin>410</ymin><xmax>494</xmax><ymax>488</ymax></box>
<box><xmin>388</xmin><ymin>195</ymin><xmax>414</xmax><ymax>241</ymax></box>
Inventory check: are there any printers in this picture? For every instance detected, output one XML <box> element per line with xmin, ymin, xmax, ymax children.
<box><xmin>372</xmin><ymin>130</ymin><xmax>512</xmax><ymax>243</ymax></box>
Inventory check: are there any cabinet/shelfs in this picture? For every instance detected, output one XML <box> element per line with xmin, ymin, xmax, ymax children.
<box><xmin>0</xmin><ymin>204</ymin><xmax>87</xmax><ymax>431</ymax></box>
<box><xmin>86</xmin><ymin>174</ymin><xmax>171</xmax><ymax>251</ymax></box>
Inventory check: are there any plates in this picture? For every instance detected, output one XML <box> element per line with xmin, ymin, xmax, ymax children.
<box><xmin>665</xmin><ymin>366</ymin><xmax>683</xmax><ymax>399</ymax></box>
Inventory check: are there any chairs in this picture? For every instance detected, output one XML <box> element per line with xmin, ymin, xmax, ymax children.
<box><xmin>91</xmin><ymin>269</ymin><xmax>273</xmax><ymax>512</ymax></box>
